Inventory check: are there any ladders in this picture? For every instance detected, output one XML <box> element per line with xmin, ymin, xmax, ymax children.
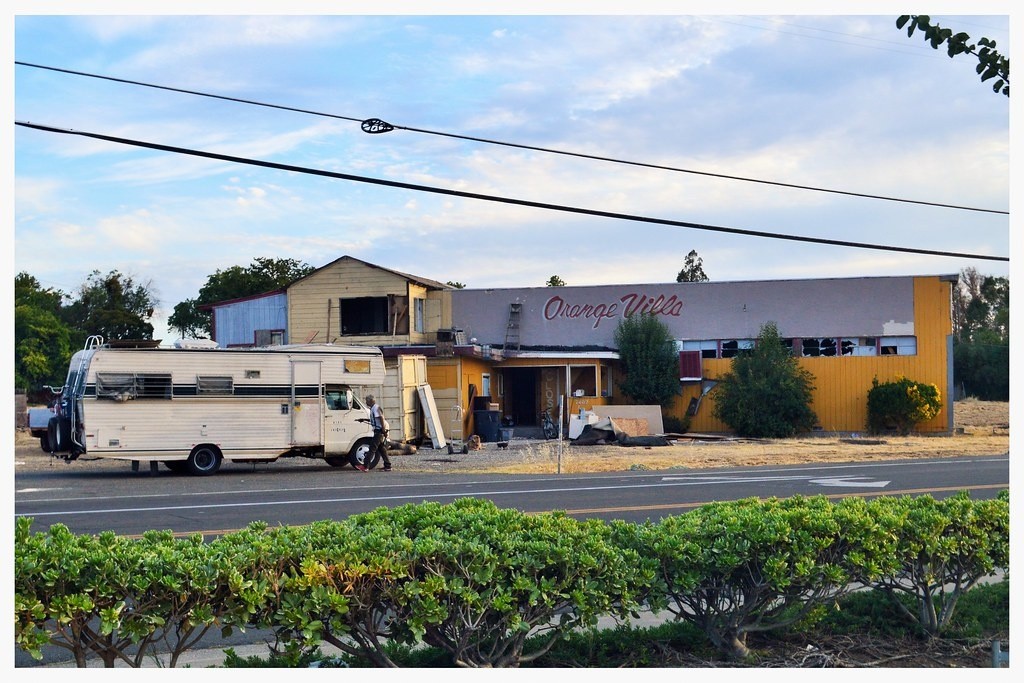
<box><xmin>503</xmin><ymin>303</ymin><xmax>523</xmax><ymax>352</ymax></box>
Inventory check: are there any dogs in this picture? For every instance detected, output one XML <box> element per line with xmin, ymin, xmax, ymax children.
<box><xmin>467</xmin><ymin>435</ymin><xmax>486</xmax><ymax>452</ymax></box>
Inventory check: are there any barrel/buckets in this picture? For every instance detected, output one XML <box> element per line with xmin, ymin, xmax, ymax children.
<box><xmin>474</xmin><ymin>410</ymin><xmax>502</xmax><ymax>443</ymax></box>
<box><xmin>501</xmin><ymin>429</ymin><xmax>513</xmax><ymax>441</ymax></box>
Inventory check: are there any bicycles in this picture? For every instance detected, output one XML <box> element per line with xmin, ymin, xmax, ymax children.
<box><xmin>537</xmin><ymin>407</ymin><xmax>558</xmax><ymax>440</ymax></box>
<box><xmin>43</xmin><ymin>385</ymin><xmax>70</xmax><ymax>451</ymax></box>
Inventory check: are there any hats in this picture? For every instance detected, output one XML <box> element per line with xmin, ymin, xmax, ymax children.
<box><xmin>366</xmin><ymin>394</ymin><xmax>376</xmax><ymax>401</ymax></box>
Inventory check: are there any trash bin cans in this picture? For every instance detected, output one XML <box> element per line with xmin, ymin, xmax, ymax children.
<box><xmin>473</xmin><ymin>409</ymin><xmax>503</xmax><ymax>443</ymax></box>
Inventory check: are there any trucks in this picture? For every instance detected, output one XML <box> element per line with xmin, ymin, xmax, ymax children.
<box><xmin>43</xmin><ymin>335</ymin><xmax>385</xmax><ymax>474</ymax></box>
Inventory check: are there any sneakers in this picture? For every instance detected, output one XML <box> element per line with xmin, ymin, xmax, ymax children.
<box><xmin>376</xmin><ymin>467</ymin><xmax>392</xmax><ymax>472</ymax></box>
<box><xmin>355</xmin><ymin>465</ymin><xmax>369</xmax><ymax>473</ymax></box>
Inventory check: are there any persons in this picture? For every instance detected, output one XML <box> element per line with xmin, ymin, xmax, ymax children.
<box><xmin>355</xmin><ymin>395</ymin><xmax>392</xmax><ymax>472</ymax></box>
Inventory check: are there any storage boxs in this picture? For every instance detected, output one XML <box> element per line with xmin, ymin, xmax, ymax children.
<box><xmin>486</xmin><ymin>401</ymin><xmax>499</xmax><ymax>411</ymax></box>
<box><xmin>576</xmin><ymin>390</ymin><xmax>585</xmax><ymax>396</ymax></box>
<box><xmin>579</xmin><ymin>408</ymin><xmax>585</xmax><ymax>419</ymax></box>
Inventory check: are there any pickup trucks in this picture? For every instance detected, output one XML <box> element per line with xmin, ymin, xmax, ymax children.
<box><xmin>26</xmin><ymin>408</ymin><xmax>56</xmax><ymax>452</ymax></box>
